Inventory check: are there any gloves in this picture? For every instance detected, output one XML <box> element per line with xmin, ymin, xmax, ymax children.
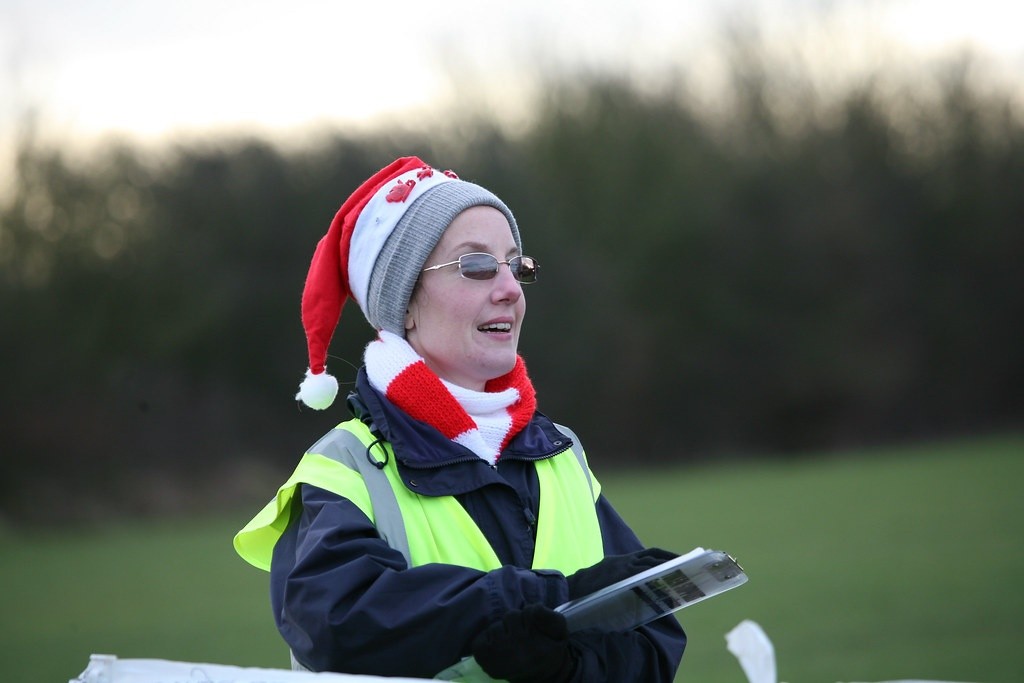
<box><xmin>472</xmin><ymin>602</ymin><xmax>575</xmax><ymax>683</ymax></box>
<box><xmin>574</xmin><ymin>548</ymin><xmax>680</xmax><ymax>596</ymax></box>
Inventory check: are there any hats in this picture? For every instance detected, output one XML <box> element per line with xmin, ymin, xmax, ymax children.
<box><xmin>294</xmin><ymin>156</ymin><xmax>459</xmax><ymax>411</ymax></box>
<box><xmin>368</xmin><ymin>180</ymin><xmax>522</xmax><ymax>341</ymax></box>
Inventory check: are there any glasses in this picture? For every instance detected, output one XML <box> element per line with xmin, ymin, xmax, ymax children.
<box><xmin>422</xmin><ymin>252</ymin><xmax>540</xmax><ymax>284</ymax></box>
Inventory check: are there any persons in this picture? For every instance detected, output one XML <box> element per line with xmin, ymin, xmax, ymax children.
<box><xmin>233</xmin><ymin>156</ymin><xmax>687</xmax><ymax>683</ymax></box>
